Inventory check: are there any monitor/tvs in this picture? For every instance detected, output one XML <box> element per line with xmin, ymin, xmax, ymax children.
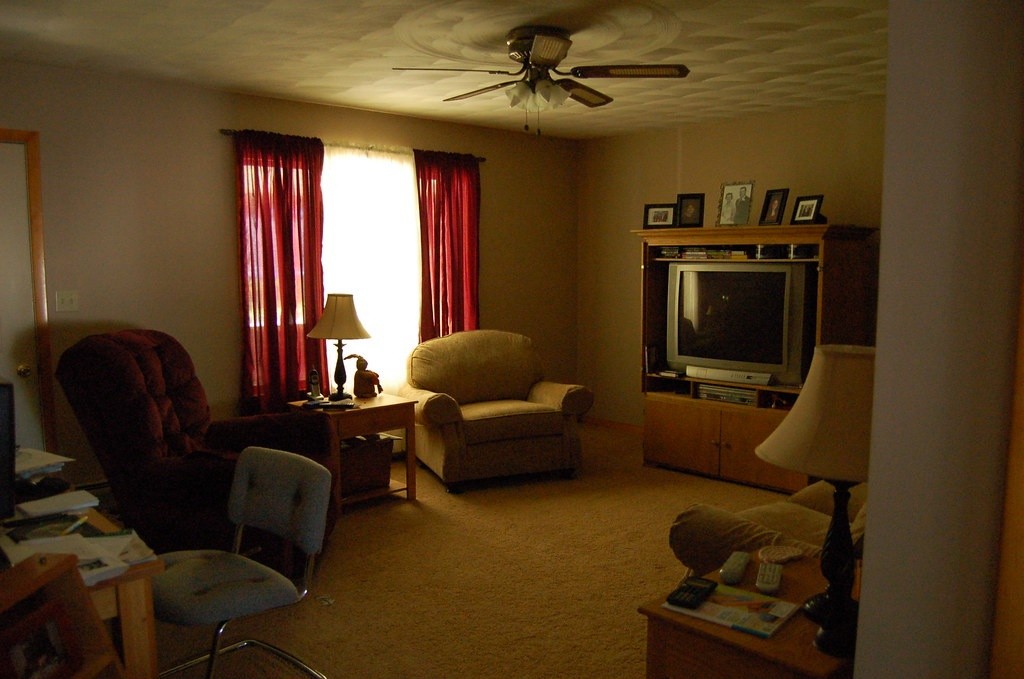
<box><xmin>0</xmin><ymin>382</ymin><xmax>16</xmax><ymax>518</ymax></box>
<box><xmin>665</xmin><ymin>262</ymin><xmax>812</xmax><ymax>383</ymax></box>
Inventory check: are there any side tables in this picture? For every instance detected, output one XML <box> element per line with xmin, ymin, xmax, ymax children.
<box><xmin>287</xmin><ymin>394</ymin><xmax>419</xmax><ymax>517</ymax></box>
<box><xmin>637</xmin><ymin>560</ymin><xmax>861</xmax><ymax>679</ymax></box>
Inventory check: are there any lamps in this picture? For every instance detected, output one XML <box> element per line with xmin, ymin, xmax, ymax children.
<box><xmin>506</xmin><ymin>69</ymin><xmax>570</xmax><ymax>137</ymax></box>
<box><xmin>306</xmin><ymin>294</ymin><xmax>370</xmax><ymax>400</ymax></box>
<box><xmin>756</xmin><ymin>344</ymin><xmax>875</xmax><ymax>621</ymax></box>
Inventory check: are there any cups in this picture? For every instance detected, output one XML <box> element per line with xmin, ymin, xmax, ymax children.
<box><xmin>787</xmin><ymin>244</ymin><xmax>808</xmax><ymax>259</ymax></box>
<box><xmin>756</xmin><ymin>244</ymin><xmax>774</xmax><ymax>258</ymax></box>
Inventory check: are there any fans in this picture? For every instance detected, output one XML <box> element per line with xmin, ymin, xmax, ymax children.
<box><xmin>392</xmin><ymin>26</ymin><xmax>691</xmax><ymax>108</ymax></box>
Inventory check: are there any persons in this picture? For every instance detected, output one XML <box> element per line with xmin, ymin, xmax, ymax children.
<box><xmin>721</xmin><ymin>192</ymin><xmax>736</xmax><ymax>224</ymax></box>
<box><xmin>765</xmin><ymin>198</ymin><xmax>780</xmax><ymax>221</ymax></box>
<box><xmin>734</xmin><ymin>187</ymin><xmax>750</xmax><ymax>224</ymax></box>
<box><xmin>681</xmin><ymin>199</ymin><xmax>699</xmax><ymax>223</ymax></box>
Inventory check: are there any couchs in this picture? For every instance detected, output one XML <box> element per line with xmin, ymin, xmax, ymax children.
<box><xmin>54</xmin><ymin>327</ymin><xmax>339</xmax><ymax>583</ymax></box>
<box><xmin>670</xmin><ymin>478</ymin><xmax>867</xmax><ymax>570</ymax></box>
<box><xmin>392</xmin><ymin>329</ymin><xmax>594</xmax><ymax>491</ymax></box>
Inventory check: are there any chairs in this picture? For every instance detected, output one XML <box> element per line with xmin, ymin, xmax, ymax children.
<box><xmin>150</xmin><ymin>446</ymin><xmax>330</xmax><ymax>679</ymax></box>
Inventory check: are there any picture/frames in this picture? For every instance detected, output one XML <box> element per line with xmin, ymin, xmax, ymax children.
<box><xmin>714</xmin><ymin>181</ymin><xmax>753</xmax><ymax>228</ymax></box>
<box><xmin>790</xmin><ymin>194</ymin><xmax>827</xmax><ymax>224</ymax></box>
<box><xmin>675</xmin><ymin>192</ymin><xmax>705</xmax><ymax>228</ymax></box>
<box><xmin>758</xmin><ymin>189</ymin><xmax>789</xmax><ymax>225</ymax></box>
<box><xmin>643</xmin><ymin>203</ymin><xmax>678</xmax><ymax>229</ymax></box>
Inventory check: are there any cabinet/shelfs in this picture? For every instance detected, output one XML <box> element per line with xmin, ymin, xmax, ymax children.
<box><xmin>629</xmin><ymin>224</ymin><xmax>880</xmax><ymax>496</ymax></box>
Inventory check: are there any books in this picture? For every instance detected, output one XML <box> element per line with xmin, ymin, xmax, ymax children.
<box><xmin>15</xmin><ymin>489</ymin><xmax>100</xmax><ymax>519</ymax></box>
<box><xmin>651</xmin><ymin>245</ymin><xmax>747</xmax><ymax>260</ymax></box>
<box><xmin>660</xmin><ymin>582</ymin><xmax>802</xmax><ymax>640</ymax></box>
<box><xmin>19</xmin><ymin>528</ymin><xmax>157</xmax><ymax>586</ymax></box>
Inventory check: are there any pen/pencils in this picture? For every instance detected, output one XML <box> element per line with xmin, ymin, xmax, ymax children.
<box><xmin>60</xmin><ymin>515</ymin><xmax>90</xmax><ymax>538</ymax></box>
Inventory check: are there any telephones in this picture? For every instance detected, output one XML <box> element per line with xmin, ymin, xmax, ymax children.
<box><xmin>306</xmin><ymin>365</ymin><xmax>324</xmax><ymax>401</ymax></box>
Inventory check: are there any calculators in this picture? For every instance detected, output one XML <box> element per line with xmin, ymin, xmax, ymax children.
<box><xmin>666</xmin><ymin>577</ymin><xmax>718</xmax><ymax>609</ymax></box>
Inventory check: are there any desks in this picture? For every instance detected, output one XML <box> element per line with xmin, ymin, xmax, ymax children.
<box><xmin>0</xmin><ymin>510</ymin><xmax>156</xmax><ymax>679</ymax></box>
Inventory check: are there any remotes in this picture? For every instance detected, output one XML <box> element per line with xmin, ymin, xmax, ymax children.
<box><xmin>303</xmin><ymin>399</ymin><xmax>354</xmax><ymax>410</ymax></box>
<box><xmin>722</xmin><ymin>550</ymin><xmax>750</xmax><ymax>584</ymax></box>
<box><xmin>756</xmin><ymin>563</ymin><xmax>783</xmax><ymax>593</ymax></box>
